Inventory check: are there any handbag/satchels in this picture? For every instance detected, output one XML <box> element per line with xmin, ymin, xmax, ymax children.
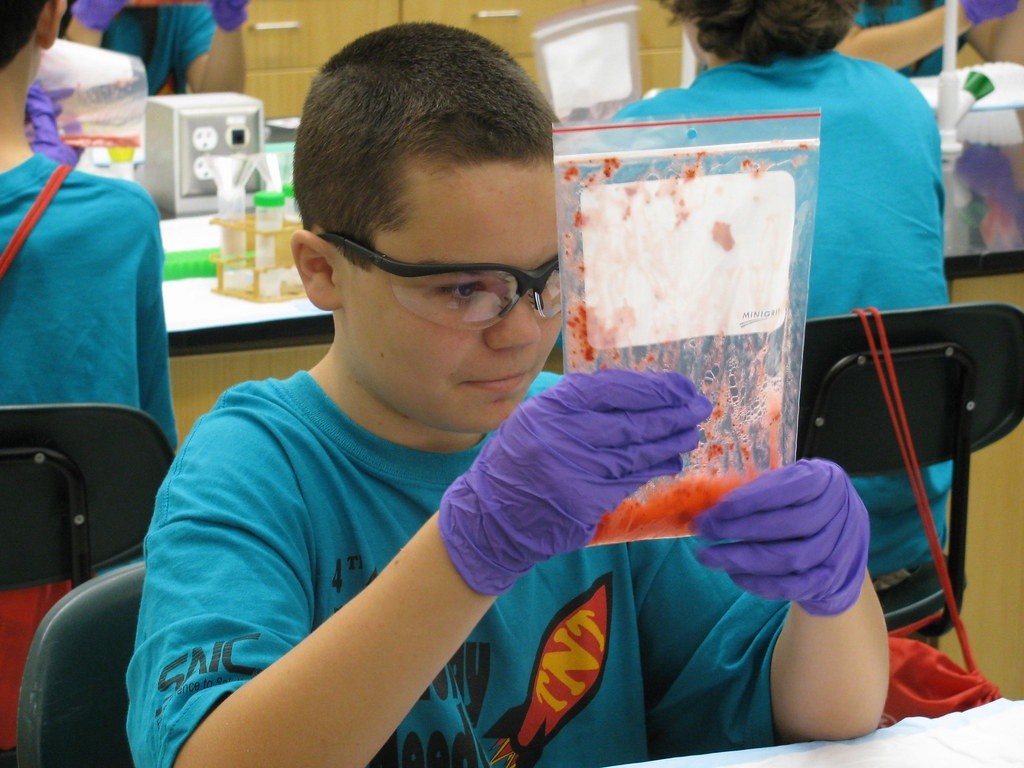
<box><xmin>877</xmin><ymin>637</ymin><xmax>997</xmax><ymax>728</ymax></box>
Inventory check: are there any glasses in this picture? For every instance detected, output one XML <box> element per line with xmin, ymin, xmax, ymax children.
<box><xmin>316</xmin><ymin>229</ymin><xmax>561</xmax><ymax>332</ymax></box>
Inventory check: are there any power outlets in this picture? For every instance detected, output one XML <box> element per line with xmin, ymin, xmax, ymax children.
<box><xmin>180</xmin><ymin>109</ymin><xmax>263</xmax><ymax>197</ymax></box>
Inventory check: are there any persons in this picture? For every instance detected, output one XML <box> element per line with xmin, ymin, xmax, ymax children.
<box><xmin>0</xmin><ymin>0</ymin><xmax>1018</xmax><ymax>748</ymax></box>
<box><xmin>127</xmin><ymin>22</ymin><xmax>889</xmax><ymax>768</ymax></box>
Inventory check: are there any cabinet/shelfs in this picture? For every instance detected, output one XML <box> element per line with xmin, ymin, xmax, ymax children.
<box><xmin>242</xmin><ymin>0</ymin><xmax>685</xmax><ymax>143</ymax></box>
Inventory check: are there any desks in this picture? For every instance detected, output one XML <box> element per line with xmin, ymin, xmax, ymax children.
<box><xmin>0</xmin><ymin>199</ymin><xmax>1024</xmax><ymax>700</ymax></box>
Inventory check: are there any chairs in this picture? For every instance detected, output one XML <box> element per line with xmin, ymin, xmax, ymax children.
<box><xmin>795</xmin><ymin>303</ymin><xmax>1024</xmax><ymax>645</ymax></box>
<box><xmin>18</xmin><ymin>561</ymin><xmax>146</xmax><ymax>768</ymax></box>
<box><xmin>0</xmin><ymin>403</ymin><xmax>174</xmax><ymax>768</ymax></box>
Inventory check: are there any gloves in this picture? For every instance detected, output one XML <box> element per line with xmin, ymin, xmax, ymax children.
<box><xmin>72</xmin><ymin>0</ymin><xmax>127</xmax><ymax>30</ymax></box>
<box><xmin>699</xmin><ymin>461</ymin><xmax>870</xmax><ymax>616</ymax></box>
<box><xmin>436</xmin><ymin>367</ymin><xmax>711</xmax><ymax>595</ymax></box>
<box><xmin>214</xmin><ymin>0</ymin><xmax>247</xmax><ymax>30</ymax></box>
<box><xmin>958</xmin><ymin>1</ymin><xmax>1017</xmax><ymax>26</ymax></box>
<box><xmin>25</xmin><ymin>82</ymin><xmax>83</xmax><ymax>168</ymax></box>
